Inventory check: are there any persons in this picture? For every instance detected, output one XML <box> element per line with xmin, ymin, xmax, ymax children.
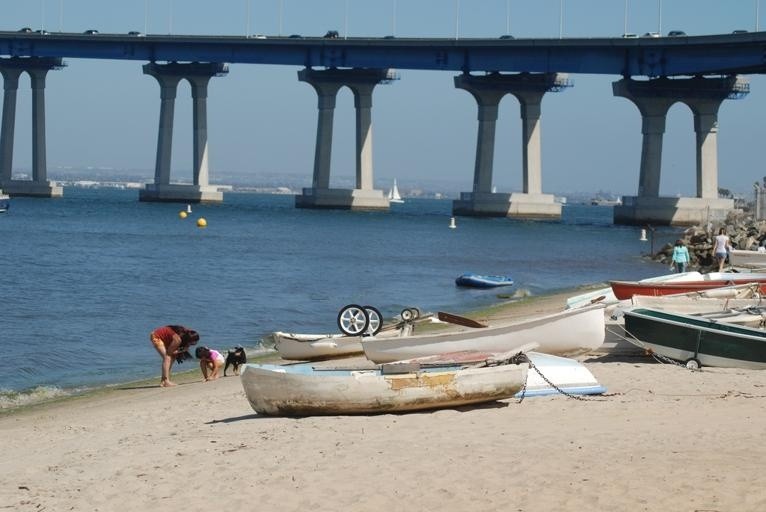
<box><xmin>712</xmin><ymin>227</ymin><xmax>732</xmax><ymax>274</ymax></box>
<box><xmin>195</xmin><ymin>346</ymin><xmax>226</xmax><ymax>382</ymax></box>
<box><xmin>669</xmin><ymin>239</ymin><xmax>691</xmax><ymax>272</ymax></box>
<box><xmin>149</xmin><ymin>324</ymin><xmax>199</xmax><ymax>386</ymax></box>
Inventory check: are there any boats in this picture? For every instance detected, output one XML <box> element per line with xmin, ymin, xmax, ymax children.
<box><xmin>236</xmin><ymin>351</ymin><xmax>529</xmax><ymax>418</ymax></box>
<box><xmin>357</xmin><ymin>299</ymin><xmax>612</xmax><ymax>369</ymax></box>
<box><xmin>609</xmin><ymin>262</ymin><xmax>765</xmax><ymax>373</ymax></box>
<box><xmin>269</xmin><ymin>326</ymin><xmax>411</xmax><ymax>364</ymax></box>
<box><xmin>454</xmin><ymin>273</ymin><xmax>514</xmax><ymax>289</ymax></box>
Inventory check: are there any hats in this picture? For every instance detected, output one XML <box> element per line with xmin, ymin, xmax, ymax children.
<box><xmin>228</xmin><ymin>343</ymin><xmax>244</xmax><ymax>353</ymax></box>
